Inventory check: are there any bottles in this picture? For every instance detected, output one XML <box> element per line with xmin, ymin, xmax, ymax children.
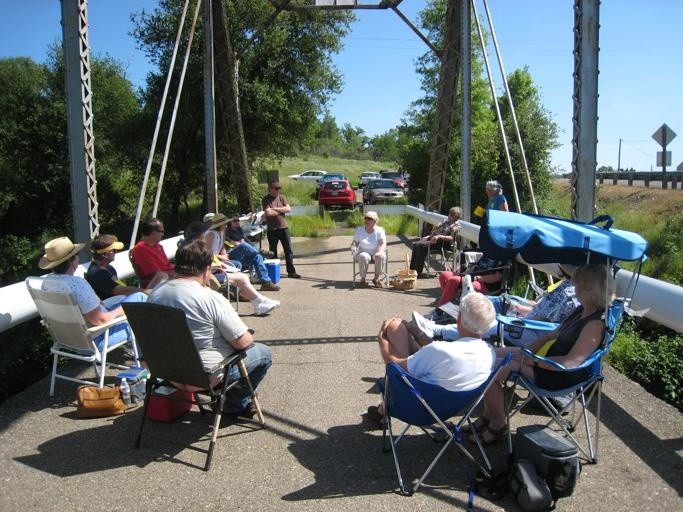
<box><xmin>119</xmin><ymin>378</ymin><xmax>131</xmax><ymax>405</ymax></box>
<box><xmin>351</xmin><ymin>242</ymin><xmax>355</xmax><ymax>256</ymax></box>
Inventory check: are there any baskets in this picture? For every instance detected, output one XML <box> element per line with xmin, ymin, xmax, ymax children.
<box><xmin>394</xmin><ymin>252</ymin><xmax>419</xmax><ymax>289</ymax></box>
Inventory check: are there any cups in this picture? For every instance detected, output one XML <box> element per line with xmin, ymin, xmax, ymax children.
<box><xmin>507</xmin><ymin>321</ymin><xmax>524</xmax><ymax>339</ymax></box>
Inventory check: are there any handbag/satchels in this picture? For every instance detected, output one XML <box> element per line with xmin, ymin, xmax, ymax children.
<box><xmin>74</xmin><ymin>382</ymin><xmax>127</xmax><ymax>418</ymax></box>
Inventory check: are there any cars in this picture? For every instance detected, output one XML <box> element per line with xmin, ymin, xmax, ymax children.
<box><xmin>285</xmin><ymin>169</ymin><xmax>328</xmax><ymax>182</ymax></box>
<box><xmin>316</xmin><ymin>178</ymin><xmax>358</xmax><ymax>210</ymax></box>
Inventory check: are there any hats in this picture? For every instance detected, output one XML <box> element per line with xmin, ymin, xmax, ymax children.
<box><xmin>90</xmin><ymin>237</ymin><xmax>126</xmax><ymax>255</ymax></box>
<box><xmin>203</xmin><ymin>212</ymin><xmax>231</xmax><ymax>230</ymax></box>
<box><xmin>35</xmin><ymin>237</ymin><xmax>86</xmax><ymax>270</ymax></box>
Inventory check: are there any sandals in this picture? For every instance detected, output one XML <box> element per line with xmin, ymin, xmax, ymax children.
<box><xmin>468</xmin><ymin>424</ymin><xmax>509</xmax><ymax>447</ymax></box>
<box><xmin>464</xmin><ymin>413</ymin><xmax>490</xmax><ymax>433</ymax></box>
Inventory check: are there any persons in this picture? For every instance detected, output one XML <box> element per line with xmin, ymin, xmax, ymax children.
<box><xmin>39</xmin><ymin>212</ymin><xmax>283</xmax><ymax>423</ymax></box>
<box><xmin>409</xmin><ymin>206</ymin><xmax>461</xmax><ymax>278</ymax></box>
<box><xmin>261</xmin><ymin>180</ymin><xmax>300</xmax><ymax>278</ymax></box>
<box><xmin>349</xmin><ymin>211</ymin><xmax>388</xmax><ymax>289</ymax></box>
<box><xmin>481</xmin><ymin>179</ymin><xmax>509</xmax><ymax>212</ymax></box>
<box><xmin>378</xmin><ymin>251</ymin><xmax>617</xmax><ymax>448</ymax></box>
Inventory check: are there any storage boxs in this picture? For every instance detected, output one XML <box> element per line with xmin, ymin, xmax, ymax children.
<box><xmin>252</xmin><ymin>258</ymin><xmax>280</xmax><ymax>285</ymax></box>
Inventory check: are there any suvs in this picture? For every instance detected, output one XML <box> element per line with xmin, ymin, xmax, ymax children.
<box><xmin>313</xmin><ymin>173</ymin><xmax>346</xmax><ymax>199</ymax></box>
<box><xmin>356</xmin><ymin>168</ymin><xmax>409</xmax><ymax>204</ymax></box>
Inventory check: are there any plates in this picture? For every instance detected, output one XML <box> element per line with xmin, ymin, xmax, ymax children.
<box><xmin>102</xmin><ymin>294</ymin><xmax>126</xmax><ymax>307</ymax></box>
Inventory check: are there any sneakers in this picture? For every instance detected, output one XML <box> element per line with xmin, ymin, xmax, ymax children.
<box><xmin>288</xmin><ymin>271</ymin><xmax>302</xmax><ymax>279</ymax></box>
<box><xmin>359</xmin><ymin>280</ymin><xmax>368</xmax><ymax>289</ymax></box>
<box><xmin>243</xmin><ymin>388</ymin><xmax>259</xmax><ymax>418</ymax></box>
<box><xmin>254</xmin><ymin>295</ymin><xmax>282</xmax><ymax>316</ymax></box>
<box><xmin>260</xmin><ymin>280</ymin><xmax>282</xmax><ymax>292</ymax></box>
<box><xmin>259</xmin><ymin>248</ymin><xmax>275</xmax><ymax>259</ymax></box>
<box><xmin>410</xmin><ymin>310</ymin><xmax>437</xmax><ymax>340</ymax></box>
<box><xmin>367</xmin><ymin>404</ymin><xmax>391</xmax><ymax>429</ymax></box>
<box><xmin>372</xmin><ymin>278</ymin><xmax>383</xmax><ymax>288</ymax></box>
<box><xmin>422</xmin><ymin>308</ymin><xmax>445</xmax><ymax>323</ymax></box>
